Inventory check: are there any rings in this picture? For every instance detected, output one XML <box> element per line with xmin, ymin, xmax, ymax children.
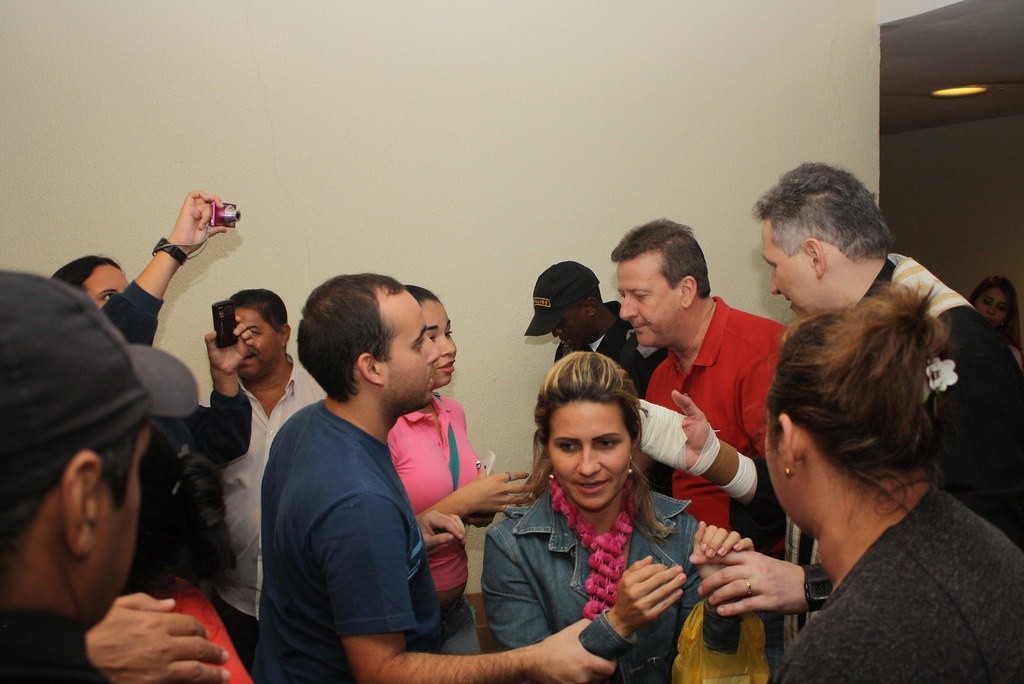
<box><xmin>746</xmin><ymin>579</ymin><xmax>751</xmax><ymax>594</ymax></box>
<box><xmin>505</xmin><ymin>472</ymin><xmax>511</xmax><ymax>480</ymax></box>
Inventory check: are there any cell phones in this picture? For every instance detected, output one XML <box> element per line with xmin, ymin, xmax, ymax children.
<box><xmin>212</xmin><ymin>299</ymin><xmax>239</xmax><ymax>347</ymax></box>
<box><xmin>482</xmin><ymin>449</ymin><xmax>495</xmax><ymax>477</ymax></box>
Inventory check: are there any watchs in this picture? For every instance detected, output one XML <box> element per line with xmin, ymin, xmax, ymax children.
<box><xmin>801</xmin><ymin>564</ymin><xmax>833</xmax><ymax>611</ymax></box>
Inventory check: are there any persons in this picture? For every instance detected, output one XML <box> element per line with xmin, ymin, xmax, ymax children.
<box><xmin>0</xmin><ymin>164</ymin><xmax>1024</xmax><ymax>684</ymax></box>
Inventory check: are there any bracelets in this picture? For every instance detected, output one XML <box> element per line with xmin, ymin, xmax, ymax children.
<box><xmin>154</xmin><ymin>238</ymin><xmax>186</xmax><ymax>265</ymax></box>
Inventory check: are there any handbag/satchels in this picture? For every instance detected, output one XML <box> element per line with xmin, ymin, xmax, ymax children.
<box><xmin>669</xmin><ymin>598</ymin><xmax>770</xmax><ymax>684</ymax></box>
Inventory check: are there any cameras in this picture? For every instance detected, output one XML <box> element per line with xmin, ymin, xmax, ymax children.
<box><xmin>209</xmin><ymin>202</ymin><xmax>240</xmax><ymax>228</ymax></box>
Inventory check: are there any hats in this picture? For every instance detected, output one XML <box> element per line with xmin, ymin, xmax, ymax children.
<box><xmin>524</xmin><ymin>262</ymin><xmax>600</xmax><ymax>337</ymax></box>
<box><xmin>1</xmin><ymin>269</ymin><xmax>201</xmax><ymax>464</ymax></box>
